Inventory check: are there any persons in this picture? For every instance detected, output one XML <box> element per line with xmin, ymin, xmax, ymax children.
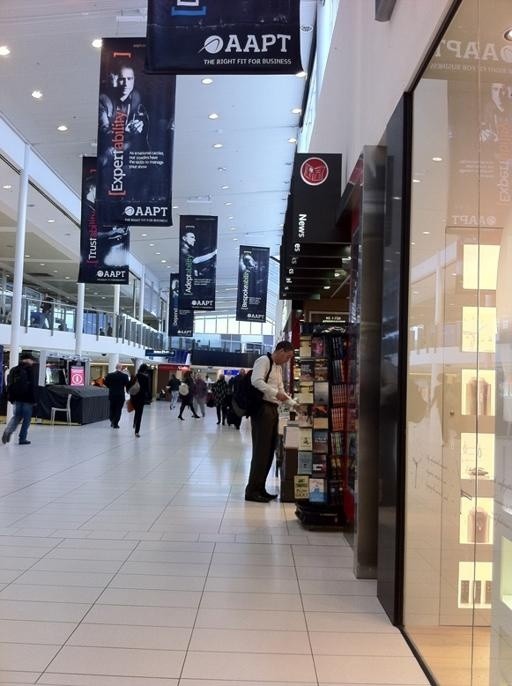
<box><xmin>211</xmin><ymin>369</ymin><xmax>246</xmax><ymax>429</ymax></box>
<box><xmin>245</xmin><ymin>341</ymin><xmax>304</xmax><ymax>502</ymax></box>
<box><xmin>105</xmin><ymin>363</ymin><xmax>129</xmax><ymax>428</ymax></box>
<box><xmin>2</xmin><ymin>354</ymin><xmax>38</xmax><ymax>444</ymax></box>
<box><xmin>39</xmin><ymin>292</ymin><xmax>53</xmax><ymax>329</ymax></box>
<box><xmin>127</xmin><ymin>364</ymin><xmax>150</xmax><ymax>437</ymax></box>
<box><xmin>180</xmin><ymin>228</ymin><xmax>217</xmax><ymax>279</ymax></box>
<box><xmin>100</xmin><ymin>323</ymin><xmax>112</xmax><ymax>336</ymax></box>
<box><xmin>82</xmin><ymin>175</ymin><xmax>127</xmax><ymax>267</ymax></box>
<box><xmin>243</xmin><ymin>250</ymin><xmax>265</xmax><ymax>272</ymax></box>
<box><xmin>379</xmin><ymin>358</ymin><xmax>398</xmax><ymax>507</ymax></box>
<box><xmin>99</xmin><ymin>60</ymin><xmax>148</xmax><ymax>183</ymax></box>
<box><xmin>167</xmin><ymin>370</ymin><xmax>207</xmax><ymax>420</ymax></box>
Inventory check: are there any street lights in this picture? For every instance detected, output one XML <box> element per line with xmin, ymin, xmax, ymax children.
<box><xmin>126</xmin><ymin>399</ymin><xmax>134</xmax><ymax>413</ymax></box>
<box><xmin>127</xmin><ymin>376</ymin><xmax>140</xmax><ymax>395</ymax></box>
<box><xmin>207</xmin><ymin>399</ymin><xmax>216</xmax><ymax>408</ymax></box>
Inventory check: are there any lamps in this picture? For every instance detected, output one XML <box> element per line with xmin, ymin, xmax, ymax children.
<box><xmin>51</xmin><ymin>394</ymin><xmax>72</xmax><ymax>426</ymax></box>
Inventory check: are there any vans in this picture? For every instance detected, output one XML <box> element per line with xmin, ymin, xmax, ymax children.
<box><xmin>192</xmin><ymin>414</ymin><xmax>200</xmax><ymax>419</ymax></box>
<box><xmin>177</xmin><ymin>415</ymin><xmax>185</xmax><ymax>420</ymax></box>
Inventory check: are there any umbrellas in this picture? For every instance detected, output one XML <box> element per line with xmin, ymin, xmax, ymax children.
<box><xmin>2</xmin><ymin>434</ymin><xmax>10</xmax><ymax>444</ymax></box>
<box><xmin>19</xmin><ymin>438</ymin><xmax>31</xmax><ymax>444</ymax></box>
<box><xmin>245</xmin><ymin>487</ymin><xmax>278</xmax><ymax>503</ymax></box>
<box><xmin>110</xmin><ymin>423</ymin><xmax>120</xmax><ymax>428</ymax></box>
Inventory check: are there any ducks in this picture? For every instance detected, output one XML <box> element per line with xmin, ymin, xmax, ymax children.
<box><xmin>227</xmin><ymin>408</ymin><xmax>236</xmax><ymax>426</ymax></box>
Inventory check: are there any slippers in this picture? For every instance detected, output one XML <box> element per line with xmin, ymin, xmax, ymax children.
<box><xmin>228</xmin><ymin>352</ymin><xmax>273</xmax><ymax>416</ymax></box>
<box><xmin>178</xmin><ymin>382</ymin><xmax>190</xmax><ymax>396</ymax></box>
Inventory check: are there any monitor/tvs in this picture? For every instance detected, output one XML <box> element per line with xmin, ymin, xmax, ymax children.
<box><xmin>294</xmin><ymin>333</ymin><xmax>347</xmax><ymax>503</ymax></box>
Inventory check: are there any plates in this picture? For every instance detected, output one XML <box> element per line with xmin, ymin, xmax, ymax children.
<box><xmin>440</xmin><ymin>227</ymin><xmax>501</xmax><ymax>628</ymax></box>
<box><xmin>280</xmin><ymin>321</ymin><xmax>358</xmax><ymax>528</ymax></box>
